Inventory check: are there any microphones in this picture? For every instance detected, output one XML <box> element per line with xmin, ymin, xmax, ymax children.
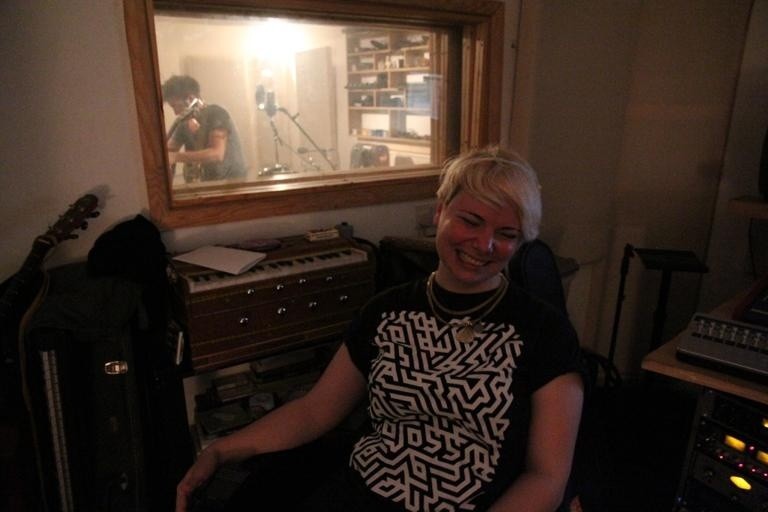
<box><xmin>266</xmin><ymin>92</ymin><xmax>275</xmax><ymax>117</ymax></box>
<box><xmin>255</xmin><ymin>84</ymin><xmax>264</xmax><ymax>110</ymax></box>
<box><xmin>297</xmin><ymin>147</ymin><xmax>335</xmax><ymax>154</ymax></box>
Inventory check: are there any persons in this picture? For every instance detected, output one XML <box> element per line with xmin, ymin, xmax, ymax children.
<box><xmin>162</xmin><ymin>73</ymin><xmax>246</xmax><ymax>184</ymax></box>
<box><xmin>175</xmin><ymin>146</ymin><xmax>597</xmax><ymax>512</ymax></box>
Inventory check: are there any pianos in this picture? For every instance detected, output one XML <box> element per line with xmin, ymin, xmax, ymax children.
<box><xmin>163</xmin><ymin>226</ymin><xmax>386</xmax><ymax>374</ymax></box>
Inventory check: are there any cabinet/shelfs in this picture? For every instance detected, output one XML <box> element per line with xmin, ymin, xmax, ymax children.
<box><xmin>341</xmin><ymin>27</ymin><xmax>433</xmax><ymax>146</ymax></box>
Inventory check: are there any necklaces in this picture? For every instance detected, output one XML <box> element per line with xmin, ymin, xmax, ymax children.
<box><xmin>429</xmin><ymin>270</ymin><xmax>505</xmax><ymax>315</ymax></box>
<box><xmin>424</xmin><ymin>279</ymin><xmax>510</xmax><ymax>343</ymax></box>
